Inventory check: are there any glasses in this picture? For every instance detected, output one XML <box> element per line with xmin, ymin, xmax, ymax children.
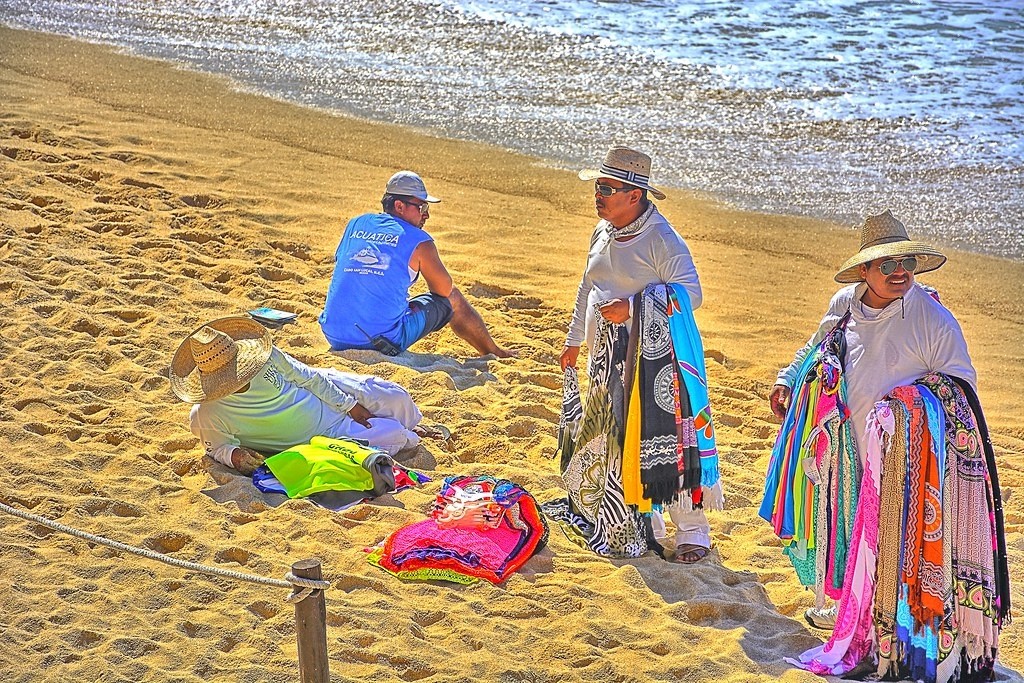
<box><xmin>870</xmin><ymin>258</ymin><xmax>918</xmax><ymax>276</ymax></box>
<box><xmin>595</xmin><ymin>181</ymin><xmax>634</xmax><ymax>198</ymax></box>
<box><xmin>401</xmin><ymin>199</ymin><xmax>430</xmax><ymax>215</ymax></box>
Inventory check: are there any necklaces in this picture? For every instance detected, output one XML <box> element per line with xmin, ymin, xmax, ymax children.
<box><xmin>859</xmin><ymin>286</ymin><xmax>900</xmax><ymax>321</ymax></box>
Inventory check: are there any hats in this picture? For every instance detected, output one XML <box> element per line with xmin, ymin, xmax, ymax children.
<box><xmin>169</xmin><ymin>316</ymin><xmax>272</xmax><ymax>404</ymax></box>
<box><xmin>833</xmin><ymin>207</ymin><xmax>948</xmax><ymax>283</ymax></box>
<box><xmin>385</xmin><ymin>170</ymin><xmax>441</xmax><ymax>203</ymax></box>
<box><xmin>578</xmin><ymin>146</ymin><xmax>666</xmax><ymax>200</ymax></box>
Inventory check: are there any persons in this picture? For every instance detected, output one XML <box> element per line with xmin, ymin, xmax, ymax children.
<box><xmin>168</xmin><ymin>314</ymin><xmax>452</xmax><ymax>479</ymax></box>
<box><xmin>559</xmin><ymin>144</ymin><xmax>714</xmax><ymax>563</ymax></box>
<box><xmin>767</xmin><ymin>214</ymin><xmax>978</xmax><ymax>636</ymax></box>
<box><xmin>318</xmin><ymin>168</ymin><xmax>515</xmax><ymax>358</ymax></box>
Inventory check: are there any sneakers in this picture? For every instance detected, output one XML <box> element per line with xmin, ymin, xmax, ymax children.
<box><xmin>804</xmin><ymin>605</ymin><xmax>838</xmax><ymax>630</ymax></box>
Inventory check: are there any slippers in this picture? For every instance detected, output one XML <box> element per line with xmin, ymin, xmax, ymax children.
<box><xmin>417</xmin><ymin>423</ymin><xmax>451</xmax><ymax>441</ymax></box>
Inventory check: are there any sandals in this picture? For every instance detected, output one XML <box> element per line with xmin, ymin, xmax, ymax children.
<box><xmin>673</xmin><ymin>543</ymin><xmax>707</xmax><ymax>564</ymax></box>
